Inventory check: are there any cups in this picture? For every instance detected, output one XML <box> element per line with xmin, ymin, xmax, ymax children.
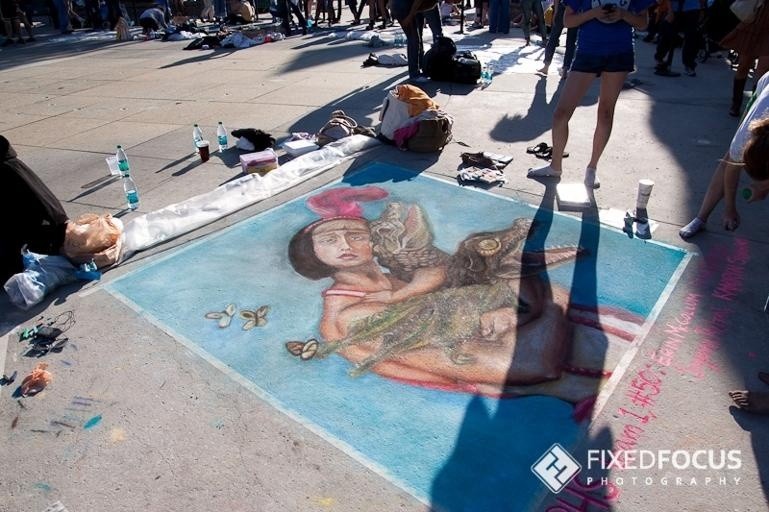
<box><xmin>105</xmin><ymin>157</ymin><xmax>120</xmax><ymax>175</ymax></box>
<box><xmin>636</xmin><ymin>178</ymin><xmax>654</xmax><ymax>210</ymax></box>
<box><xmin>196</xmin><ymin>140</ymin><xmax>210</xmax><ymax>161</ymax></box>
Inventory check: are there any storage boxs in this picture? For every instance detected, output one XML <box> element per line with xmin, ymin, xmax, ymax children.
<box><xmin>239</xmin><ymin>150</ymin><xmax>278</xmax><ymax>176</ymax></box>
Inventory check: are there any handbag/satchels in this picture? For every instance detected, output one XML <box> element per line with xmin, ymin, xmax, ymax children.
<box><xmin>729</xmin><ymin>0</ymin><xmax>763</xmax><ymax>24</ymax></box>
<box><xmin>421</xmin><ymin>35</ymin><xmax>481</xmax><ymax>86</ymax></box>
<box><xmin>320</xmin><ymin>109</ymin><xmax>358</xmax><ymax>138</ymax></box>
<box><xmin>379</xmin><ymin>83</ymin><xmax>452</xmax><ymax>153</ymax></box>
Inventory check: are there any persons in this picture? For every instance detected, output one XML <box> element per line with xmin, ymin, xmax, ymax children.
<box><xmin>526</xmin><ymin>0</ymin><xmax>650</xmax><ymax>188</ymax></box>
<box><xmin>1</xmin><ymin>1</ymin><xmax>768</xmax><ymax>117</ymax></box>
<box><xmin>678</xmin><ymin>72</ymin><xmax>769</xmax><ymax>238</ymax></box>
<box><xmin>728</xmin><ymin>371</ymin><xmax>769</xmax><ymax>414</ymax></box>
<box><xmin>280</xmin><ymin>212</ymin><xmax>645</xmax><ymax>422</ymax></box>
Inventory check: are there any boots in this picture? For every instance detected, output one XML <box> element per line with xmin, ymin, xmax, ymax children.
<box><xmin>728</xmin><ymin>78</ymin><xmax>747</xmax><ymax>118</ymax></box>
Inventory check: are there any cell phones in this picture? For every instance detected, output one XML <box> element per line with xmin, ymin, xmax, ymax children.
<box><xmin>602</xmin><ymin>3</ymin><xmax>617</xmax><ymax>13</ymax></box>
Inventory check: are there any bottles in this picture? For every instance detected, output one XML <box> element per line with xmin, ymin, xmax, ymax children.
<box><xmin>394</xmin><ymin>32</ymin><xmax>408</xmax><ymax>47</ymax></box>
<box><xmin>123</xmin><ymin>172</ymin><xmax>139</xmax><ymax>210</ymax></box>
<box><xmin>217</xmin><ymin>122</ymin><xmax>228</xmax><ymax>152</ymax></box>
<box><xmin>482</xmin><ymin>63</ymin><xmax>494</xmax><ymax>83</ymax></box>
<box><xmin>193</xmin><ymin>124</ymin><xmax>204</xmax><ymax>154</ymax></box>
<box><xmin>116</xmin><ymin>145</ymin><xmax>130</xmax><ymax>177</ymax></box>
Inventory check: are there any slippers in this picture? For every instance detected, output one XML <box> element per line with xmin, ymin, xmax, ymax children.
<box><xmin>527</xmin><ymin>142</ymin><xmax>571</xmax><ymax>159</ymax></box>
